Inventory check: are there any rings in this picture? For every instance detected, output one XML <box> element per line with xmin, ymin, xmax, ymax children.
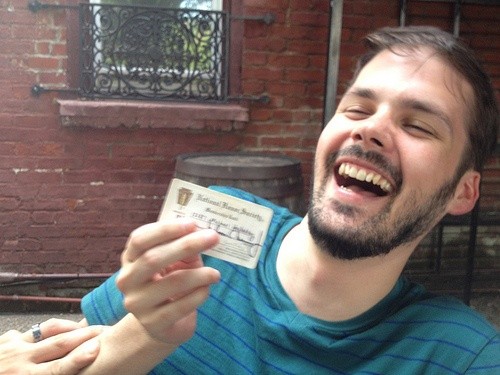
<box><xmin>31</xmin><ymin>323</ymin><xmax>42</xmax><ymax>343</ymax></box>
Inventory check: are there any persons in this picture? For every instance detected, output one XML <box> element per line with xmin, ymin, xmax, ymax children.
<box><xmin>0</xmin><ymin>317</ymin><xmax>104</xmax><ymax>375</ymax></box>
<box><xmin>76</xmin><ymin>25</ymin><xmax>500</xmax><ymax>375</ymax></box>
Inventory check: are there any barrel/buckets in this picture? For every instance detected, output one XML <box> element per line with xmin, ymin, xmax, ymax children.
<box><xmin>173</xmin><ymin>151</ymin><xmax>305</xmax><ymax>217</ymax></box>
